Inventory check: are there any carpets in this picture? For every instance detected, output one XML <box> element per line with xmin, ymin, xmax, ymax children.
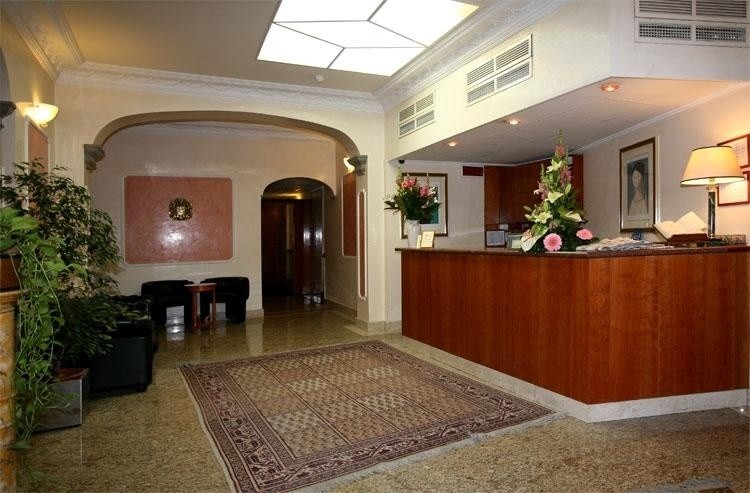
<box><xmin>176</xmin><ymin>340</ymin><xmax>558</xmax><ymax>492</ymax></box>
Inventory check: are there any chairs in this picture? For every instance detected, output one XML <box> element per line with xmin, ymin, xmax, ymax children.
<box><xmin>199</xmin><ymin>277</ymin><xmax>250</xmax><ymax>329</ymax></box>
<box><xmin>141</xmin><ymin>279</ymin><xmax>193</xmax><ymax>331</ymax></box>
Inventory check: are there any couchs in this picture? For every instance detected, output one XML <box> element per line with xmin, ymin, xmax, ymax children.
<box><xmin>67</xmin><ymin>295</ymin><xmax>160</xmax><ymax>398</ymax></box>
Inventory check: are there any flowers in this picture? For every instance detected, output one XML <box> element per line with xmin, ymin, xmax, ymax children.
<box><xmin>521</xmin><ymin>126</ymin><xmax>600</xmax><ymax>252</ymax></box>
<box><xmin>383</xmin><ymin>172</ymin><xmax>446</xmax><ymax>220</ymax></box>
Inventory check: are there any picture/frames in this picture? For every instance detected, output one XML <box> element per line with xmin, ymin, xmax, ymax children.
<box><xmin>717</xmin><ymin>170</ymin><xmax>750</xmax><ymax>206</ymax></box>
<box><xmin>716</xmin><ymin>134</ymin><xmax>750</xmax><ymax>168</ymax></box>
<box><xmin>619</xmin><ymin>136</ymin><xmax>659</xmax><ymax>231</ymax></box>
<box><xmin>401</xmin><ymin>172</ymin><xmax>449</xmax><ymax>238</ymax></box>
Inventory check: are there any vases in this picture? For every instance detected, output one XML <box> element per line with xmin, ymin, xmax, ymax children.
<box><xmin>406</xmin><ymin>220</ymin><xmax>421</xmax><ymax>247</ymax></box>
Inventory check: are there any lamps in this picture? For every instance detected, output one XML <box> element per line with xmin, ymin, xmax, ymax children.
<box><xmin>680</xmin><ymin>146</ymin><xmax>740</xmax><ymax>241</ymax></box>
<box><xmin>16</xmin><ymin>102</ymin><xmax>60</xmax><ymax>128</ymax></box>
<box><xmin>255</xmin><ymin>1</ymin><xmax>480</xmax><ymax>78</ymax></box>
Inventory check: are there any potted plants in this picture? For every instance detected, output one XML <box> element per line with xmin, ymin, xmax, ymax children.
<box><xmin>1</xmin><ymin>158</ymin><xmax>122</xmax><ymax>453</ymax></box>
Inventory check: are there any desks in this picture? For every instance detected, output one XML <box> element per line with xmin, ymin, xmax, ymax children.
<box><xmin>182</xmin><ymin>282</ymin><xmax>217</xmax><ymax>331</ymax></box>
